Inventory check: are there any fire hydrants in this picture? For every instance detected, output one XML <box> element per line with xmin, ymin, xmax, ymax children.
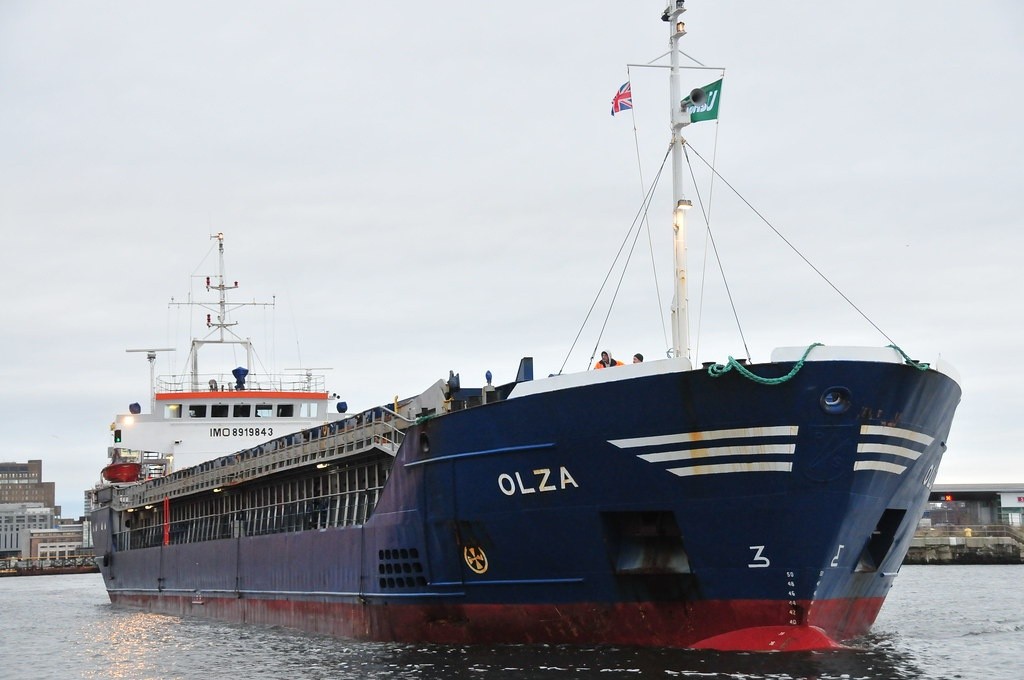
<box><xmin>964</xmin><ymin>527</ymin><xmax>972</xmax><ymax>537</ymax></box>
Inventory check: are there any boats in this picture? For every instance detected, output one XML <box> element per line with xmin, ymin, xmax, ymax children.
<box><xmin>100</xmin><ymin>461</ymin><xmax>142</xmax><ymax>482</ymax></box>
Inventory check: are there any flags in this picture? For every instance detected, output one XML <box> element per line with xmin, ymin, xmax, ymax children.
<box><xmin>612</xmin><ymin>81</ymin><xmax>632</xmax><ymax>115</ymax></box>
<box><xmin>681</xmin><ymin>79</ymin><xmax>723</xmax><ymax>128</ymax></box>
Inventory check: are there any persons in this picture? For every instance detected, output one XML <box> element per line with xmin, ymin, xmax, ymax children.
<box><xmin>592</xmin><ymin>350</ymin><xmax>627</xmax><ymax>370</ymax></box>
<box><xmin>632</xmin><ymin>353</ymin><xmax>644</xmax><ymax>364</ymax></box>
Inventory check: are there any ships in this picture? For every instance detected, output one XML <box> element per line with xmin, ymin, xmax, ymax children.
<box><xmin>77</xmin><ymin>0</ymin><xmax>963</xmax><ymax>654</ymax></box>
